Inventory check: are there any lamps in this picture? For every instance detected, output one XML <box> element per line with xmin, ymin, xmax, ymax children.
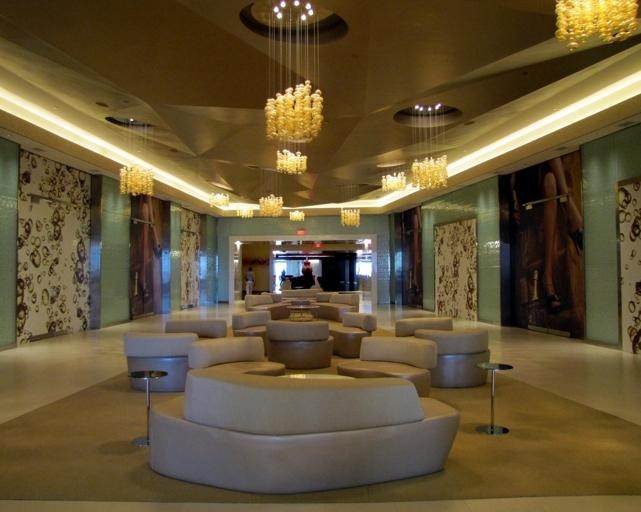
<box><xmin>379</xmin><ymin>164</ymin><xmax>410</xmax><ymax>194</ymax></box>
<box><xmin>554</xmin><ymin>0</ymin><xmax>640</xmax><ymax>56</ymax></box>
<box><xmin>271</xmin><ymin>142</ymin><xmax>311</xmax><ymax>178</ymax></box>
<box><xmin>259</xmin><ymin>1</ymin><xmax>329</xmax><ymax>146</ymax></box>
<box><xmin>338</xmin><ymin>185</ymin><xmax>364</xmax><ymax>227</ymax></box>
<box><xmin>119</xmin><ymin>118</ymin><xmax>160</xmax><ymax>199</ymax></box>
<box><xmin>410</xmin><ymin>103</ymin><xmax>454</xmax><ymax>192</ymax></box>
<box><xmin>207</xmin><ymin>179</ymin><xmax>307</xmax><ymax>223</ymax></box>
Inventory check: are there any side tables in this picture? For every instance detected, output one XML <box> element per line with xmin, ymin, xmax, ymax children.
<box><xmin>478</xmin><ymin>364</ymin><xmax>514</xmax><ymax>436</ymax></box>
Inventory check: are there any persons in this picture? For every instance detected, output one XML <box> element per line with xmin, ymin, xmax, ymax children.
<box><xmin>244</xmin><ymin>267</ymin><xmax>255</xmax><ymax>295</ymax></box>
<box><xmin>509</xmin><ymin>157</ymin><xmax>583</xmax><ymax>313</ymax></box>
<box><xmin>138</xmin><ymin>195</ymin><xmax>162</xmax><ymax>302</ymax></box>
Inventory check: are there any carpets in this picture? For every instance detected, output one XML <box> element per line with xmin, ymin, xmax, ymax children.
<box><xmin>1</xmin><ymin>320</ymin><xmax>641</xmax><ymax>504</ymax></box>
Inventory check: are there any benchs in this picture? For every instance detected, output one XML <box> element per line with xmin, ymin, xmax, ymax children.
<box><xmin>145</xmin><ymin>365</ymin><xmax>466</xmax><ymax>496</ymax></box>
<box><xmin>125</xmin><ymin>322</ymin><xmax>229</xmax><ymax>396</ymax></box>
<box><xmin>338</xmin><ymin>316</ymin><xmax>493</xmax><ymax>399</ymax></box>
<box><xmin>229</xmin><ymin>292</ymin><xmax>378</xmax><ymax>372</ymax></box>
